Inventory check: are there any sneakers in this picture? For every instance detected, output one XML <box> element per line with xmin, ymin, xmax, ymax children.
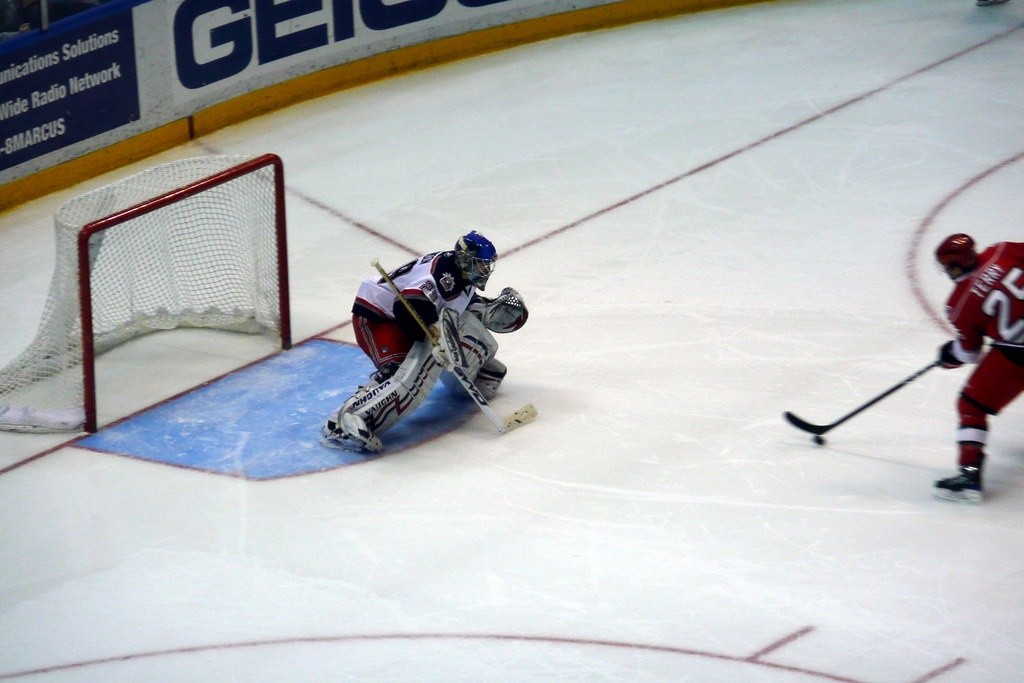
<box><xmin>929</xmin><ymin>471</ymin><xmax>985</xmax><ymax>502</ymax></box>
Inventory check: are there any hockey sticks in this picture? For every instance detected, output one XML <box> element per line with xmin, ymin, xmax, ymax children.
<box><xmin>369</xmin><ymin>256</ymin><xmax>538</xmax><ymax>435</ymax></box>
<box><xmin>782</xmin><ymin>360</ymin><xmax>943</xmax><ymax>435</ymax></box>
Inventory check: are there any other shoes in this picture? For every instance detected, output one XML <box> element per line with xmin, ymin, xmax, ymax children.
<box><xmin>322</xmin><ymin>419</ymin><xmax>364</xmax><ymax>453</ymax></box>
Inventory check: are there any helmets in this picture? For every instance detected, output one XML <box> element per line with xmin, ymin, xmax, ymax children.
<box><xmin>935</xmin><ymin>233</ymin><xmax>979</xmax><ymax>276</ymax></box>
<box><xmin>456</xmin><ymin>230</ymin><xmax>498</xmax><ymax>291</ymax></box>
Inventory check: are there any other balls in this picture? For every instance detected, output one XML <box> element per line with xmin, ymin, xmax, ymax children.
<box><xmin>814</xmin><ymin>435</ymin><xmax>823</xmax><ymax>444</ymax></box>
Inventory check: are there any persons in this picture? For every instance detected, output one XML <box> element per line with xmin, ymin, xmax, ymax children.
<box><xmin>324</xmin><ymin>229</ymin><xmax>528</xmax><ymax>453</ymax></box>
<box><xmin>932</xmin><ymin>233</ymin><xmax>1024</xmax><ymax>492</ymax></box>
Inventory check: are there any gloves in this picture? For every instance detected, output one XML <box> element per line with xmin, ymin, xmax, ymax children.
<box><xmin>936</xmin><ymin>341</ymin><xmax>964</xmax><ymax>368</ymax></box>
